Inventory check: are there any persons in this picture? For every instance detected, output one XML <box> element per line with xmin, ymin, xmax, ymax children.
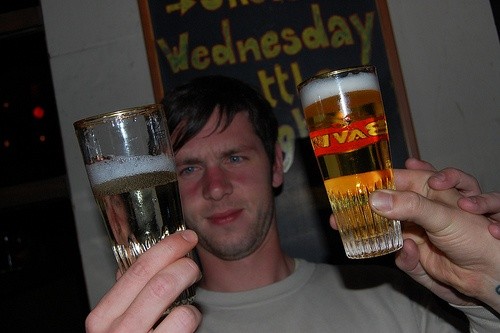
<box><xmin>85</xmin><ymin>73</ymin><xmax>500</xmax><ymax>333</ymax></box>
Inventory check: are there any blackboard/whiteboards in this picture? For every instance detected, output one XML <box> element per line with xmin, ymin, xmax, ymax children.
<box><xmin>137</xmin><ymin>0</ymin><xmax>422</xmax><ymax>281</ymax></box>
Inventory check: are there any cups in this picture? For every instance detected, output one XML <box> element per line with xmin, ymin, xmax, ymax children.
<box><xmin>74</xmin><ymin>103</ymin><xmax>194</xmax><ymax>274</ymax></box>
<box><xmin>297</xmin><ymin>66</ymin><xmax>404</xmax><ymax>260</ymax></box>
